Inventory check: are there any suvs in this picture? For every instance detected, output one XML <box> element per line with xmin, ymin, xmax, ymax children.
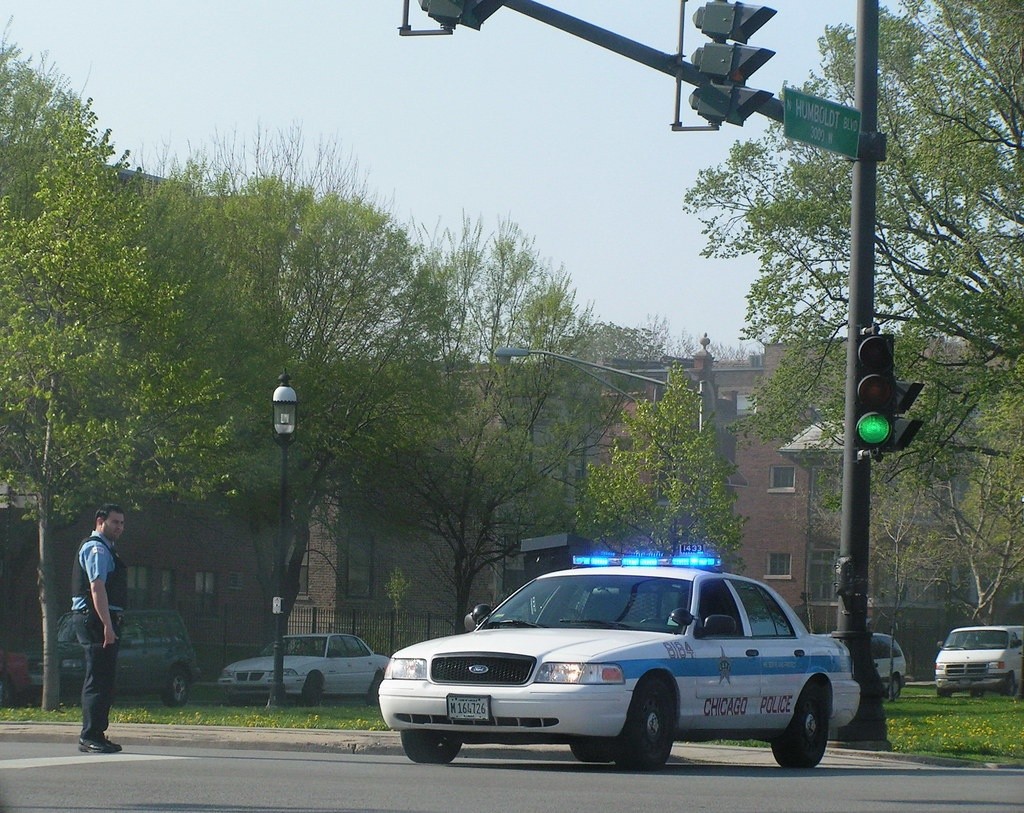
<box><xmin>811</xmin><ymin>632</ymin><xmax>907</xmax><ymax>701</ymax></box>
<box><xmin>21</xmin><ymin>608</ymin><xmax>202</xmax><ymax>707</ymax></box>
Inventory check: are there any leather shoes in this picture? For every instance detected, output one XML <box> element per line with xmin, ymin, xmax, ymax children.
<box><xmin>78</xmin><ymin>733</ymin><xmax>122</xmax><ymax>753</ymax></box>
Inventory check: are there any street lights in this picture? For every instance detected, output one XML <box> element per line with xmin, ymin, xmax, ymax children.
<box><xmin>268</xmin><ymin>366</ymin><xmax>298</xmax><ymax>707</ymax></box>
<box><xmin>496</xmin><ymin>347</ymin><xmax>709</xmax><ymax>440</ymax></box>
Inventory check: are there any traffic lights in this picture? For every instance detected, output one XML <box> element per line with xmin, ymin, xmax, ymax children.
<box><xmin>893</xmin><ymin>381</ymin><xmax>924</xmax><ymax>453</ymax></box>
<box><xmin>857</xmin><ymin>335</ymin><xmax>896</xmax><ymax>448</ymax></box>
<box><xmin>687</xmin><ymin>0</ymin><xmax>778</xmax><ymax>128</ymax></box>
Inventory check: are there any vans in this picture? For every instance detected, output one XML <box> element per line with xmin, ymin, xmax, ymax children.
<box><xmin>935</xmin><ymin>625</ymin><xmax>1024</xmax><ymax>698</ymax></box>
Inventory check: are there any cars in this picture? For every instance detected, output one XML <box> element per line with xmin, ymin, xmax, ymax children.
<box><xmin>0</xmin><ymin>625</ymin><xmax>32</xmax><ymax>701</ymax></box>
<box><xmin>217</xmin><ymin>633</ymin><xmax>390</xmax><ymax>706</ymax></box>
<box><xmin>379</xmin><ymin>553</ymin><xmax>860</xmax><ymax>772</ymax></box>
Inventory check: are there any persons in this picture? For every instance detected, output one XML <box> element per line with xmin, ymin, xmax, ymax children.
<box><xmin>71</xmin><ymin>504</ymin><xmax>127</xmax><ymax>754</ymax></box>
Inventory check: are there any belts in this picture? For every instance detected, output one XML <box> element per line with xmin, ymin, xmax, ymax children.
<box><xmin>73</xmin><ymin>609</ymin><xmax>89</xmax><ymax>615</ymax></box>
